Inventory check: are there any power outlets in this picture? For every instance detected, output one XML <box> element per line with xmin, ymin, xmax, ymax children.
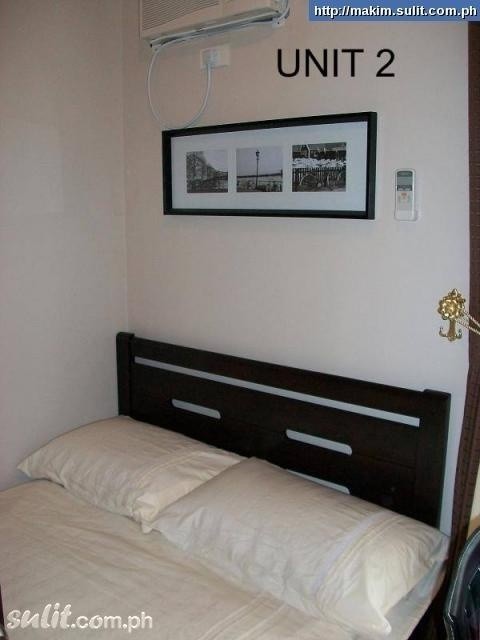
<box><xmin>198</xmin><ymin>45</ymin><xmax>229</xmax><ymax>68</ymax></box>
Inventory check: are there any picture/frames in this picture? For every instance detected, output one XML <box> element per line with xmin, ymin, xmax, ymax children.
<box><xmin>160</xmin><ymin>113</ymin><xmax>377</xmax><ymax>218</ymax></box>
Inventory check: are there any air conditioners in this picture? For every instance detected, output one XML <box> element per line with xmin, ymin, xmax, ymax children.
<box><xmin>139</xmin><ymin>0</ymin><xmax>289</xmax><ymax>46</ymax></box>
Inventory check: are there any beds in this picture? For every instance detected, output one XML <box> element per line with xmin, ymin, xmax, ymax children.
<box><xmin>0</xmin><ymin>331</ymin><xmax>451</xmax><ymax>639</ymax></box>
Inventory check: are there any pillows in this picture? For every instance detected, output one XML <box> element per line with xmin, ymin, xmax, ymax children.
<box><xmin>18</xmin><ymin>411</ymin><xmax>446</xmax><ymax>639</ymax></box>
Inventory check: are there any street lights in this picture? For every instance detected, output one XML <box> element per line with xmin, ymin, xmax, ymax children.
<box><xmin>255</xmin><ymin>148</ymin><xmax>261</xmax><ymax>190</ymax></box>
